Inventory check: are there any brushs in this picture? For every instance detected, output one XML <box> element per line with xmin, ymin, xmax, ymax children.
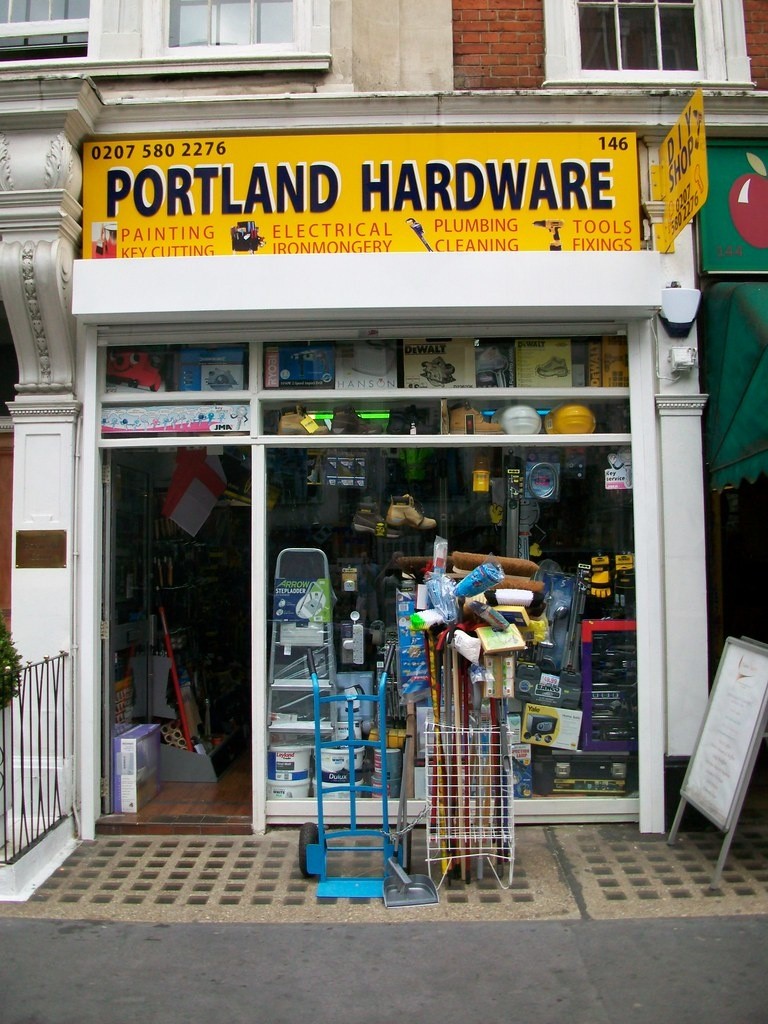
<box><xmin>411</xmin><ymin>551</ymin><xmax>544</xmax><ymax>884</ymax></box>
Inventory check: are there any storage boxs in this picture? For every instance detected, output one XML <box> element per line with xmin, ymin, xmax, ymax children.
<box><xmin>312</xmin><ymin>745</ymin><xmax>365</xmax><ymax>798</ymax></box>
<box><xmin>265</xmin><ymin>745</ymin><xmax>311</xmax><ymax>800</ymax></box>
<box><xmin>152</xmin><ymin>713</ymin><xmax>244</xmax><ymax>783</ymax></box>
<box><xmin>158</xmin><ymin>452</ymin><xmax>281</xmax><ymax>538</ymax></box>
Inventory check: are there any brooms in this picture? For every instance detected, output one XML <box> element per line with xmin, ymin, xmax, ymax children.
<box><xmin>395</xmin><ymin>538</ymin><xmax>545</xmax><ymax>885</ymax></box>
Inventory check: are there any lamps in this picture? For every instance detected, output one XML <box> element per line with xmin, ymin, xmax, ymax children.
<box><xmin>658</xmin><ymin>287</ymin><xmax>702</xmax><ymax>340</ymax></box>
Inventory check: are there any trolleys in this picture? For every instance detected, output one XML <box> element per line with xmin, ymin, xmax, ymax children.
<box><xmin>297</xmin><ymin>647</ymin><xmax>406</xmax><ymax>899</ymax></box>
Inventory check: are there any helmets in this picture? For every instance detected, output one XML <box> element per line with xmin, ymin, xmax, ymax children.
<box><xmin>491</xmin><ymin>404</ymin><xmax>542</xmax><ymax>434</ymax></box>
<box><xmin>543</xmin><ymin>401</ymin><xmax>596</xmax><ymax>434</ymax></box>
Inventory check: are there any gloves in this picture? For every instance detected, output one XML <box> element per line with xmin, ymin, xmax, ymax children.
<box><xmin>615</xmin><ymin>554</ymin><xmax>635</xmax><ymax>607</ymax></box>
<box><xmin>591</xmin><ymin>556</ymin><xmax>611</xmax><ymax>598</ymax></box>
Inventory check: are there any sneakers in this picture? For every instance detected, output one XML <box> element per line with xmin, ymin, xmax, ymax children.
<box><xmin>332</xmin><ymin>405</ymin><xmax>383</xmax><ymax>435</ymax></box>
<box><xmin>353</xmin><ymin>502</ymin><xmax>401</xmax><ymax>537</ymax></box>
<box><xmin>278</xmin><ymin>405</ymin><xmax>328</xmax><ymax>435</ymax></box>
<box><xmin>386</xmin><ymin>494</ymin><xmax>437</xmax><ymax>530</ymax></box>
<box><xmin>387</xmin><ymin>404</ymin><xmax>440</xmax><ymax>435</ymax></box>
<box><xmin>449</xmin><ymin>401</ymin><xmax>504</xmax><ymax>435</ymax></box>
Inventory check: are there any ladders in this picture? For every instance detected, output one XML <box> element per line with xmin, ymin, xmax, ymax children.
<box><xmin>264</xmin><ymin>547</ymin><xmax>339</xmax><ymax>753</ymax></box>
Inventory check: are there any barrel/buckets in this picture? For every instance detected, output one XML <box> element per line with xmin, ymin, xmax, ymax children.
<box><xmin>267</xmin><ymin>747</ymin><xmax>400</xmax><ymax>798</ymax></box>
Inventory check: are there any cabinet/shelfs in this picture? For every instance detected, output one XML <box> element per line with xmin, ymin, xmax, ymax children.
<box><xmin>151</xmin><ymin>541</ymin><xmax>207</xmax><ymax>699</ymax></box>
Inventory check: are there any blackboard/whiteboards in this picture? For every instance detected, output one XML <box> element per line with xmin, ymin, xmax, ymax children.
<box><xmin>678</xmin><ymin>636</ymin><xmax>768</xmax><ymax>834</ymax></box>
